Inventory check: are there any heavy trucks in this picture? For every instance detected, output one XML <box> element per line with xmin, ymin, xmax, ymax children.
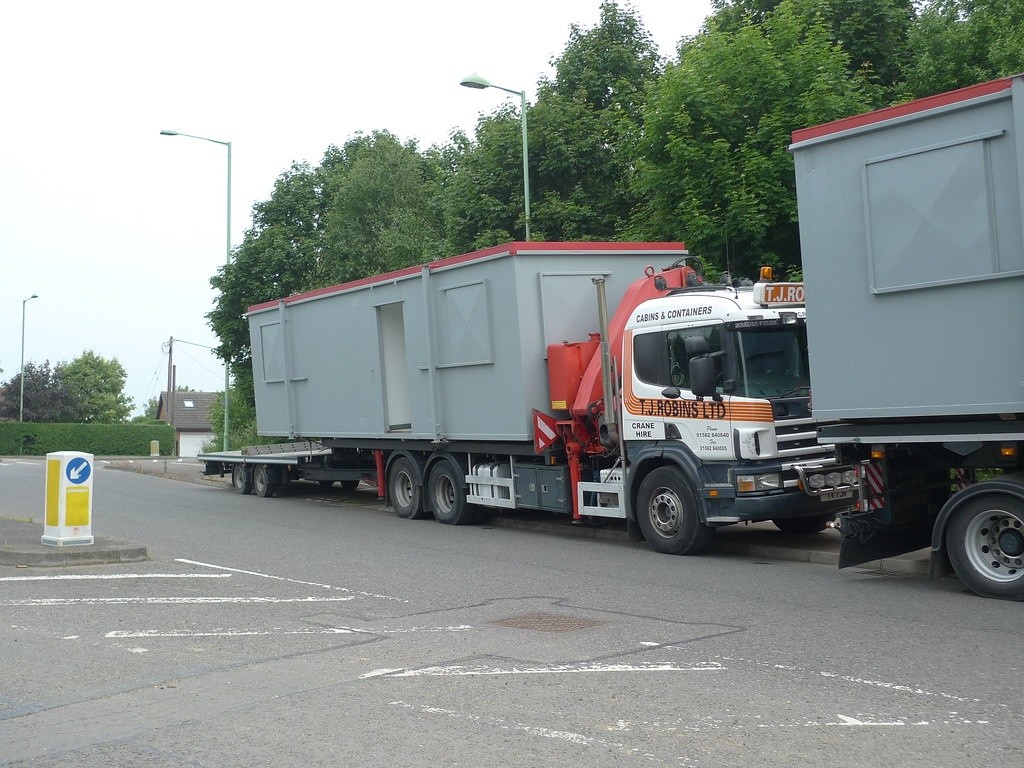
<box><xmin>194</xmin><ymin>240</ymin><xmax>873</xmax><ymax>556</ymax></box>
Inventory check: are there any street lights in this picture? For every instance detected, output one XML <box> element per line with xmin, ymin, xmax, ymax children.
<box><xmin>459</xmin><ymin>76</ymin><xmax>532</xmax><ymax>242</ymax></box>
<box><xmin>159</xmin><ymin>128</ymin><xmax>232</xmax><ymax>449</ymax></box>
<box><xmin>19</xmin><ymin>295</ymin><xmax>39</xmax><ymax>425</ymax></box>
